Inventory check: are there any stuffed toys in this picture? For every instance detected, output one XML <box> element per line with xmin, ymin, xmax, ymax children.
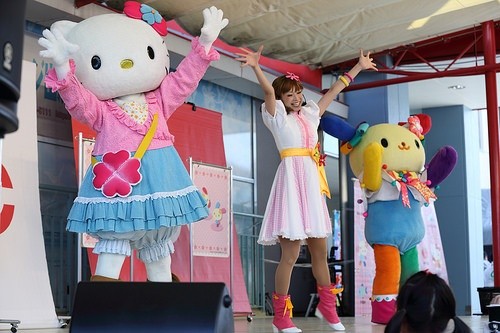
<box><xmin>37</xmin><ymin>0</ymin><xmax>230</xmax><ymax>282</ymax></box>
<box><xmin>320</xmin><ymin>112</ymin><xmax>458</xmax><ymax>325</ymax></box>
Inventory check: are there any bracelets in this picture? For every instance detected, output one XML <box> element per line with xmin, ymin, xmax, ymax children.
<box><xmin>339</xmin><ymin>72</ymin><xmax>355</xmax><ymax>86</ymax></box>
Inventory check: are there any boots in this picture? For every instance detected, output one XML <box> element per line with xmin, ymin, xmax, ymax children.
<box><xmin>315</xmin><ymin>284</ymin><xmax>345</xmax><ymax>330</ymax></box>
<box><xmin>271</xmin><ymin>291</ymin><xmax>303</xmax><ymax>333</ymax></box>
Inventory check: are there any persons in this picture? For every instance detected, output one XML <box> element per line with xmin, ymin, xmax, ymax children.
<box><xmin>233</xmin><ymin>45</ymin><xmax>378</xmax><ymax>333</ymax></box>
<box><xmin>383</xmin><ymin>269</ymin><xmax>473</xmax><ymax>333</ymax></box>
